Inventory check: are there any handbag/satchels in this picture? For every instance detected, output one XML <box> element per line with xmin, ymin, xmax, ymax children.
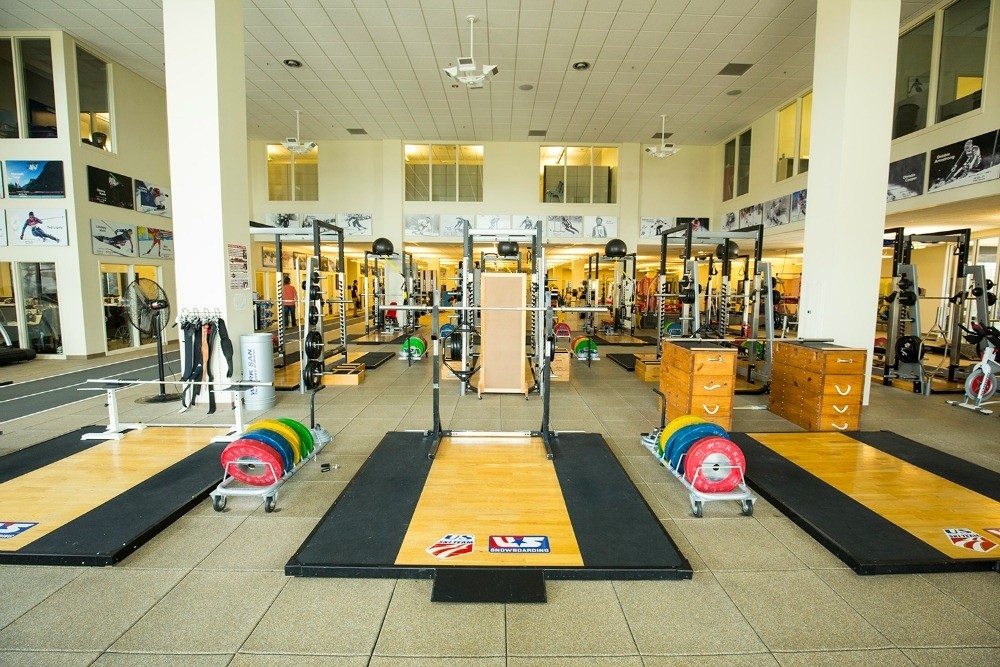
<box><xmin>356</xmin><ymin>300</ymin><xmax>361</xmax><ymax>308</ymax></box>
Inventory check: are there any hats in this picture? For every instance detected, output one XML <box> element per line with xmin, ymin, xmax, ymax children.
<box><xmin>353</xmin><ymin>280</ymin><xmax>358</xmax><ymax>282</ymax></box>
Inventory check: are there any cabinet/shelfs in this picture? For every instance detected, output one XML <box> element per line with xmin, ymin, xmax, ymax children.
<box><xmin>654</xmin><ymin>336</ymin><xmax>740</xmax><ymax>430</ymax></box>
<box><xmin>767</xmin><ymin>339</ymin><xmax>869</xmax><ymax>430</ymax></box>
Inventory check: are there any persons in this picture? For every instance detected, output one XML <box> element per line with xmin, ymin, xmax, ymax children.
<box><xmin>346</xmin><ymin>215</ymin><xmax>368</xmax><ymax>231</ymax></box>
<box><xmin>105</xmin><ymin>228</ymin><xmax>134</xmax><ymax>254</ymax></box>
<box><xmin>20</xmin><ymin>212</ymin><xmax>60</xmax><ymax>243</ymax></box>
<box><xmin>561</xmin><ymin>217</ymin><xmax>580</xmax><ymax>235</ymax></box>
<box><xmin>280</xmin><ymin>214</ymin><xmax>290</xmax><ymax>228</ymax></box>
<box><xmin>351</xmin><ymin>280</ymin><xmax>362</xmax><ymax>317</ymax></box>
<box><xmin>380</xmin><ymin>283</ymin><xmax>385</xmax><ymax>305</ymax></box>
<box><xmin>683</xmin><ymin>218</ymin><xmax>700</xmax><ymax>235</ymax></box>
<box><xmin>145</xmin><ymin>228</ymin><xmax>166</xmax><ymax>257</ymax></box>
<box><xmin>796</xmin><ymin>194</ymin><xmax>806</xmax><ymax>218</ymax></box>
<box><xmin>769</xmin><ymin>199</ymin><xmax>783</xmax><ymax>226</ymax></box>
<box><xmin>454</xmin><ymin>217</ymin><xmax>472</xmax><ymax>230</ymax></box>
<box><xmin>415</xmin><ymin>217</ymin><xmax>431</xmax><ymax>234</ymax></box>
<box><xmin>592</xmin><ymin>217</ymin><xmax>608</xmax><ymax>238</ymax></box>
<box><xmin>520</xmin><ymin>216</ymin><xmax>534</xmax><ymax>230</ymax></box>
<box><xmin>142</xmin><ymin>186</ymin><xmax>170</xmax><ymax>210</ymax></box>
<box><xmin>282</xmin><ymin>278</ymin><xmax>299</xmax><ymax>329</ymax></box>
<box><xmin>950</xmin><ymin>140</ymin><xmax>997</xmax><ymax>182</ymax></box>
<box><xmin>651</xmin><ymin>218</ymin><xmax>667</xmax><ymax>236</ymax></box>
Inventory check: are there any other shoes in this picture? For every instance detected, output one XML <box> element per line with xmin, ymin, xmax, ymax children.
<box><xmin>353</xmin><ymin>314</ymin><xmax>360</xmax><ymax>317</ymax></box>
<box><xmin>292</xmin><ymin>324</ymin><xmax>298</xmax><ymax>328</ymax></box>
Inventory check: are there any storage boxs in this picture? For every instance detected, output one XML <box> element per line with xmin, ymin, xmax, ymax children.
<box><xmin>550</xmin><ymin>347</ymin><xmax>570</xmax><ymax>381</ymax></box>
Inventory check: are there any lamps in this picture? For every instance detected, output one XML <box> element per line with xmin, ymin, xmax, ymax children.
<box><xmin>280</xmin><ymin>110</ymin><xmax>315</xmax><ymax>154</ymax></box>
<box><xmin>645</xmin><ymin>113</ymin><xmax>679</xmax><ymax>156</ymax></box>
<box><xmin>443</xmin><ymin>15</ymin><xmax>499</xmax><ymax>88</ymax></box>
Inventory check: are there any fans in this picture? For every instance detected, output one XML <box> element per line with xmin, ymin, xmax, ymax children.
<box><xmin>121</xmin><ymin>277</ymin><xmax>186</xmax><ymax>404</ymax></box>
